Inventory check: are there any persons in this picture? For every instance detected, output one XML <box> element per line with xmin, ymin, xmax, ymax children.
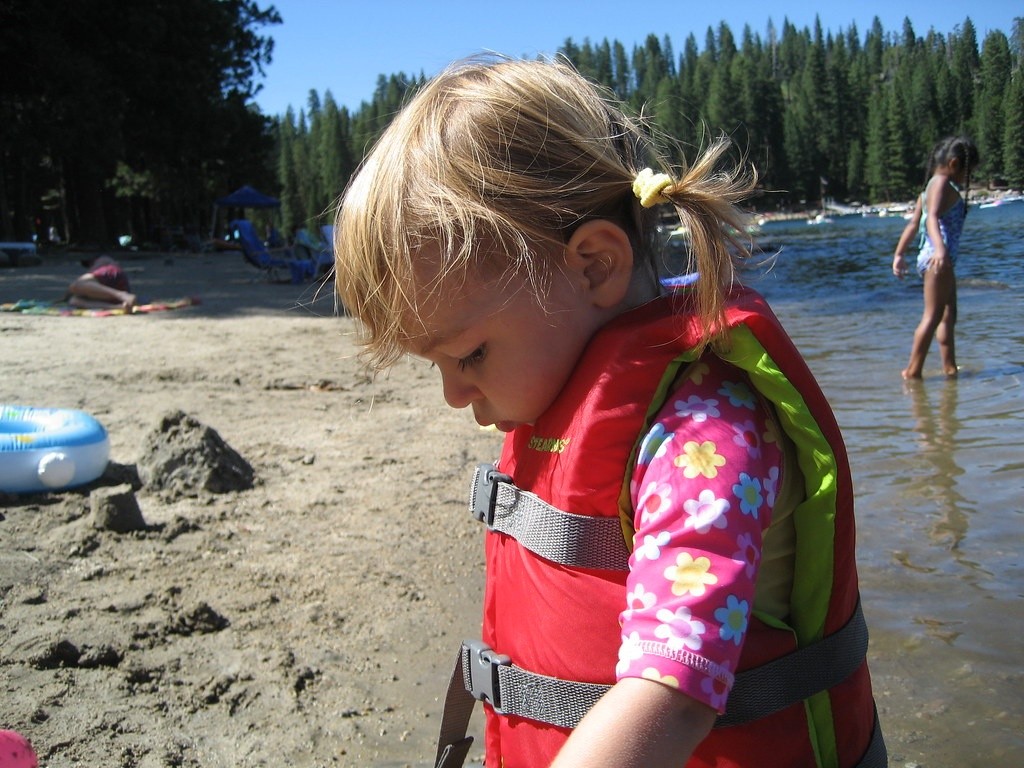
<box><xmin>893</xmin><ymin>137</ymin><xmax>979</xmax><ymax>379</ymax></box>
<box><xmin>65</xmin><ymin>253</ymin><xmax>136</xmax><ymax>313</ymax></box>
<box><xmin>331</xmin><ymin>52</ymin><xmax>889</xmax><ymax>768</ymax></box>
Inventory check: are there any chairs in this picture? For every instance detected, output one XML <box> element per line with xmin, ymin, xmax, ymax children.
<box><xmin>228</xmin><ymin>219</ymin><xmax>335</xmax><ymax>286</ymax></box>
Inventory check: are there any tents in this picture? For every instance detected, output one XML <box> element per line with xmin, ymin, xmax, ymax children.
<box><xmin>222</xmin><ymin>186</ymin><xmax>281</xmax><ymax>226</ymax></box>
